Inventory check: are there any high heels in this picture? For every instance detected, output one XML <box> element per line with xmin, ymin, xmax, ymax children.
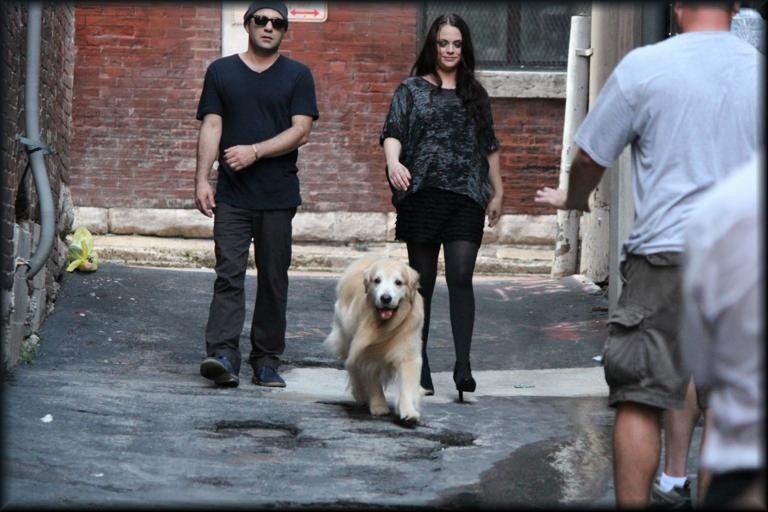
<box><xmin>454</xmin><ymin>368</ymin><xmax>476</xmax><ymax>401</ymax></box>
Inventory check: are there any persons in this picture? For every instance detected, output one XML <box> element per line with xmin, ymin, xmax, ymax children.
<box><xmin>533</xmin><ymin>3</ymin><xmax>761</xmax><ymax>507</ymax></box>
<box><xmin>191</xmin><ymin>2</ymin><xmax>324</xmax><ymax>389</ymax></box>
<box><xmin>651</xmin><ymin>370</ymin><xmax>702</xmax><ymax>506</ymax></box>
<box><xmin>378</xmin><ymin>12</ymin><xmax>506</xmax><ymax>403</ymax></box>
<box><xmin>662</xmin><ymin>154</ymin><xmax>767</xmax><ymax>510</ymax></box>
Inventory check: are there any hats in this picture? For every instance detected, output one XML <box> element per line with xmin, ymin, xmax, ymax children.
<box><xmin>244</xmin><ymin>0</ymin><xmax>289</xmax><ymax>25</ymax></box>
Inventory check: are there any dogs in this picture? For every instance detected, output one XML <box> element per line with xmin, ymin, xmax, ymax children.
<box><xmin>321</xmin><ymin>252</ymin><xmax>425</xmax><ymax>425</ymax></box>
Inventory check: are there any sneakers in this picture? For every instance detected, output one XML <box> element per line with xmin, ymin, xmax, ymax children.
<box><xmin>251</xmin><ymin>367</ymin><xmax>286</xmax><ymax>388</ymax></box>
<box><xmin>200</xmin><ymin>356</ymin><xmax>238</xmax><ymax>387</ymax></box>
<box><xmin>649</xmin><ymin>476</ymin><xmax>692</xmax><ymax>511</ymax></box>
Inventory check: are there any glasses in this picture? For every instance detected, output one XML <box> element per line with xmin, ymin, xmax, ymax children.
<box><xmin>249</xmin><ymin>15</ymin><xmax>285</xmax><ymax>29</ymax></box>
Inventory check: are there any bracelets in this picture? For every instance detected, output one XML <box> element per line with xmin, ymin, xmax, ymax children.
<box><xmin>251</xmin><ymin>143</ymin><xmax>260</xmax><ymax>161</ymax></box>
